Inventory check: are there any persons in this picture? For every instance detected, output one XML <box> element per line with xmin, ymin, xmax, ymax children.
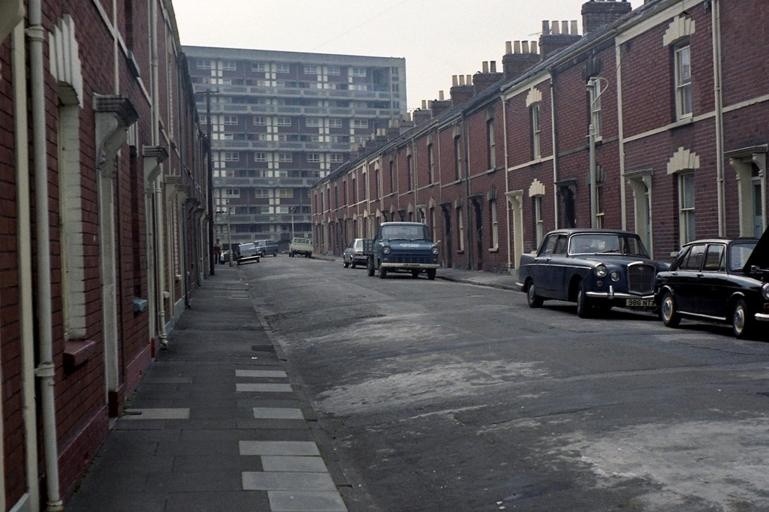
<box><xmin>213</xmin><ymin>238</ymin><xmax>221</xmax><ymax>265</ymax></box>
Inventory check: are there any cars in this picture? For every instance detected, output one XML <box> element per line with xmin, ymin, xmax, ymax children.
<box><xmin>219</xmin><ymin>240</ymin><xmax>280</xmax><ymax>264</ymax></box>
<box><xmin>654</xmin><ymin>229</ymin><xmax>766</xmax><ymax>337</ymax></box>
<box><xmin>517</xmin><ymin>230</ymin><xmax>669</xmax><ymax>316</ymax></box>
<box><xmin>342</xmin><ymin>238</ymin><xmax>371</xmax><ymax>270</ymax></box>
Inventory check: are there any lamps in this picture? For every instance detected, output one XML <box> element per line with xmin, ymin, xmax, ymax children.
<box><xmin>585</xmin><ymin>77</ymin><xmax>608</xmax><ymax>107</ymax></box>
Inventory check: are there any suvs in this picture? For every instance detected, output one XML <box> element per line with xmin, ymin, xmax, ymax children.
<box><xmin>368</xmin><ymin>222</ymin><xmax>437</xmax><ymax>280</ymax></box>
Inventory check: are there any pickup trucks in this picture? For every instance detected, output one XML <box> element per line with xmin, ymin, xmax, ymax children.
<box><xmin>288</xmin><ymin>237</ymin><xmax>314</xmax><ymax>258</ymax></box>
